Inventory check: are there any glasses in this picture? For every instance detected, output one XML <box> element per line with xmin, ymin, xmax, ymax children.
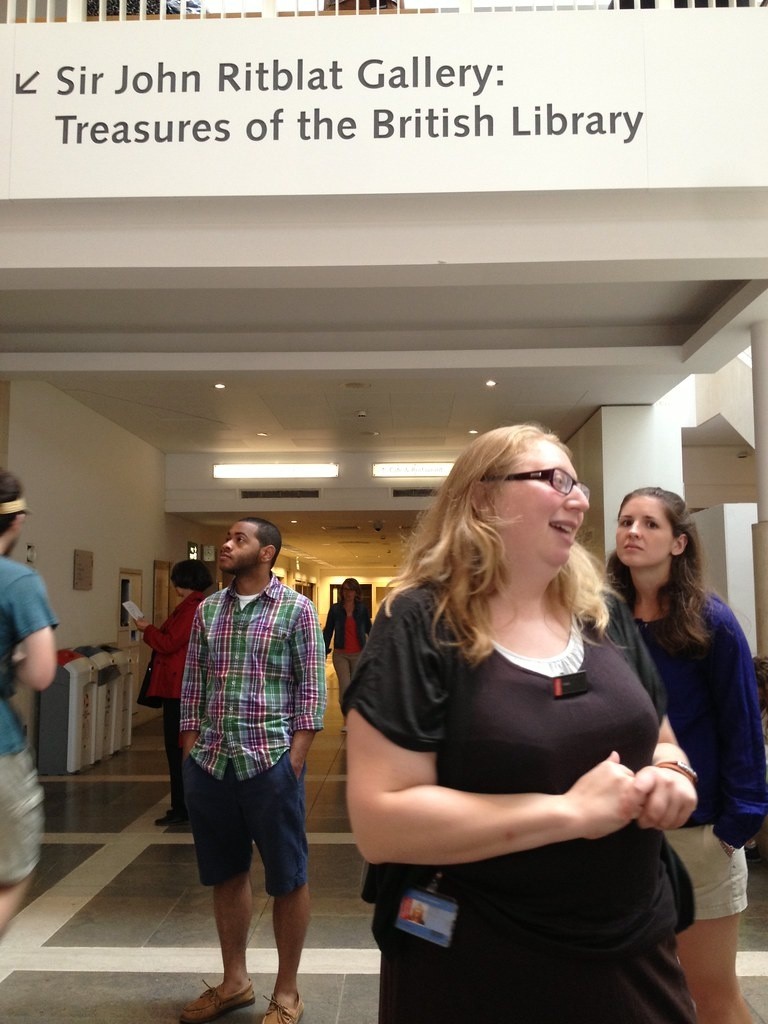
<box><xmin>481</xmin><ymin>468</ymin><xmax>591</xmax><ymax>503</ymax></box>
<box><xmin>342</xmin><ymin>587</ymin><xmax>355</xmax><ymax>591</ymax></box>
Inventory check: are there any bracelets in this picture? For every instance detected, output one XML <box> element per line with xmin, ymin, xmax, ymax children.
<box><xmin>724</xmin><ymin>842</ymin><xmax>735</xmax><ymax>852</ymax></box>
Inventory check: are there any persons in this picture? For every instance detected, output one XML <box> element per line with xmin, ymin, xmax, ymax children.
<box><xmin>180</xmin><ymin>517</ymin><xmax>327</xmax><ymax>1024</ymax></box>
<box><xmin>341</xmin><ymin>425</ymin><xmax>698</xmax><ymax>1024</ymax></box>
<box><xmin>0</xmin><ymin>467</ymin><xmax>59</xmax><ymax>928</ymax></box>
<box><xmin>605</xmin><ymin>487</ymin><xmax>768</xmax><ymax>1024</ymax></box>
<box><xmin>133</xmin><ymin>559</ymin><xmax>214</xmax><ymax>828</ymax></box>
<box><xmin>407</xmin><ymin>905</ymin><xmax>425</xmax><ymax>926</ymax></box>
<box><xmin>322</xmin><ymin>578</ymin><xmax>373</xmax><ymax>735</ymax></box>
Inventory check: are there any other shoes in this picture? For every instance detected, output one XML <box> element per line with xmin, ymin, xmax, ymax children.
<box><xmin>180</xmin><ymin>978</ymin><xmax>256</xmax><ymax>1023</ymax></box>
<box><xmin>340</xmin><ymin>727</ymin><xmax>346</xmax><ymax>735</ymax></box>
<box><xmin>261</xmin><ymin>990</ymin><xmax>304</xmax><ymax>1024</ymax></box>
<box><xmin>155</xmin><ymin>810</ymin><xmax>192</xmax><ymax>826</ymax></box>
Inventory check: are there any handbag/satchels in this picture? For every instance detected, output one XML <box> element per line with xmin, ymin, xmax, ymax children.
<box><xmin>137</xmin><ymin>666</ymin><xmax>163</xmax><ymax>708</ymax></box>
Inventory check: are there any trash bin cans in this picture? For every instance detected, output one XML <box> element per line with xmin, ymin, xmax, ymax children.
<box><xmin>73</xmin><ymin>645</ymin><xmax>122</xmax><ymax>762</ymax></box>
<box><xmin>39</xmin><ymin>649</ymin><xmax>98</xmax><ymax>775</ymax></box>
<box><xmin>98</xmin><ymin>644</ymin><xmax>133</xmax><ymax>752</ymax></box>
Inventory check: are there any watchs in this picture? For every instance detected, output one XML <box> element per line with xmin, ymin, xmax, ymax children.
<box><xmin>654</xmin><ymin>761</ymin><xmax>698</xmax><ymax>787</ymax></box>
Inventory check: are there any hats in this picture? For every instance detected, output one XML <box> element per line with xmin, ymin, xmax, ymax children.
<box><xmin>170</xmin><ymin>558</ymin><xmax>213</xmax><ymax>591</ymax></box>
<box><xmin>0</xmin><ymin>497</ymin><xmax>34</xmax><ymax>516</ymax></box>
